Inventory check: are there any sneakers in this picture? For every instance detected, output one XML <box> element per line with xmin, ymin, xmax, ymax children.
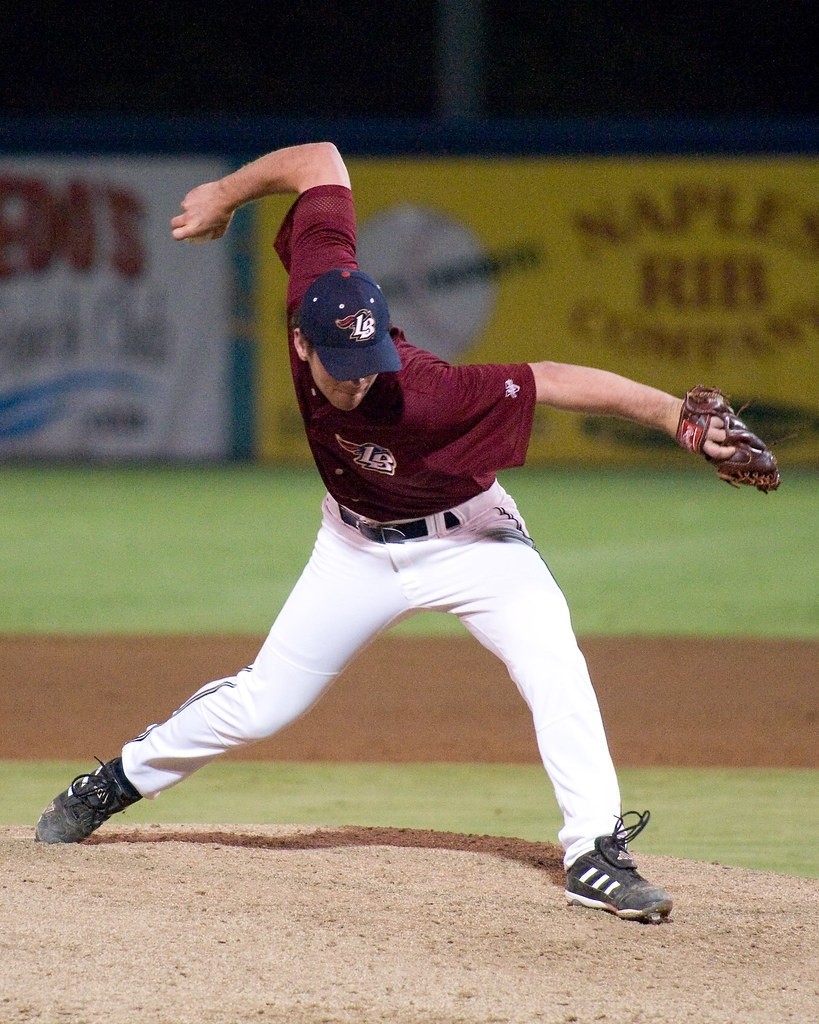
<box><xmin>564</xmin><ymin>810</ymin><xmax>675</xmax><ymax>921</ymax></box>
<box><xmin>32</xmin><ymin>755</ymin><xmax>144</xmax><ymax>846</ymax></box>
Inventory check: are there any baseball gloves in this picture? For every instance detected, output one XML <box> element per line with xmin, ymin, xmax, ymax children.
<box><xmin>674</xmin><ymin>383</ymin><xmax>781</xmax><ymax>494</ymax></box>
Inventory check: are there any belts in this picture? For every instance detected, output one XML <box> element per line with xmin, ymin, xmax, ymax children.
<box><xmin>339</xmin><ymin>505</ymin><xmax>459</xmax><ymax>544</ymax></box>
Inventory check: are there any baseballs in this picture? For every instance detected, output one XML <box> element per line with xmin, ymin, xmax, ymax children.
<box><xmin>184</xmin><ymin>230</ymin><xmax>215</xmax><ymax>244</ymax></box>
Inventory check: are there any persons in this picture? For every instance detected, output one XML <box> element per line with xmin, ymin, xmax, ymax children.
<box><xmin>35</xmin><ymin>140</ymin><xmax>736</xmax><ymax>921</ymax></box>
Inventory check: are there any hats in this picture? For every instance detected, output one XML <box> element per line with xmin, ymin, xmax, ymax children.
<box><xmin>297</xmin><ymin>268</ymin><xmax>403</xmax><ymax>383</ymax></box>
